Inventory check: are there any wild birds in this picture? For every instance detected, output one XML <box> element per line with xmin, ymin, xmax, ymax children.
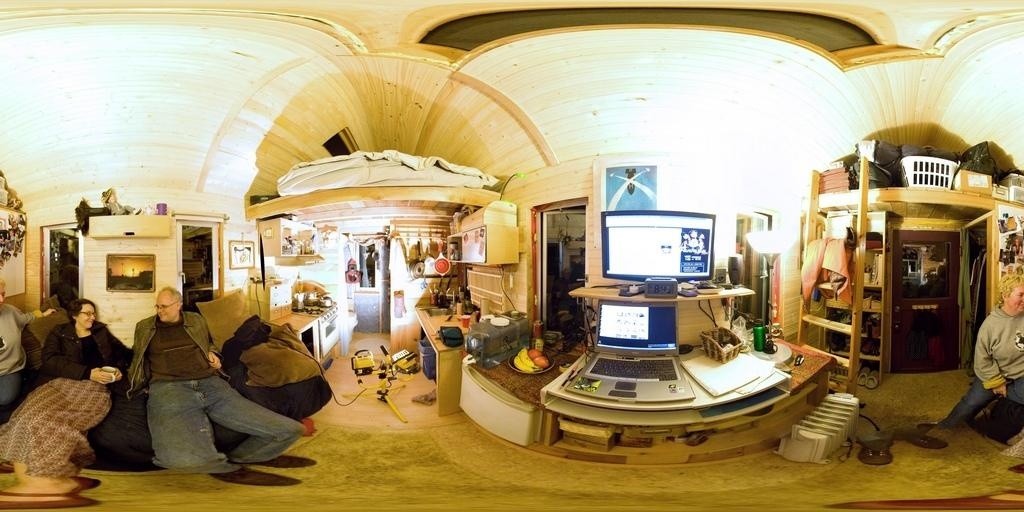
<box><xmin>565</xmin><ymin>298</ymin><xmax>696</xmax><ymax>403</ymax></box>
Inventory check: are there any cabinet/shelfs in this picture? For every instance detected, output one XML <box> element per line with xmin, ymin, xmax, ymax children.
<box><xmin>535</xmin><ymin>283</ymin><xmax>832</xmax><ymax>464</ymax></box>
<box><xmin>753</xmin><ymin>319</ymin><xmax>767</xmax><ymax>352</ymax></box>
<box><xmin>819</xmin><ymin>211</ymin><xmax>890</xmax><ymax>386</ymax></box>
<box><xmin>446</xmin><ymin>224</ymin><xmax>519</xmax><ymax>265</ymax></box>
<box><xmin>457</xmin><ymin>301</ymin><xmax>463</xmax><ymax>315</ymax></box>
<box><xmin>531</xmin><ymin>325</ymin><xmax>544</xmax><ymax>352</ymax></box>
<box><xmin>258</xmin><ymin>217</ymin><xmax>318</xmax><ymax>256</ymax></box>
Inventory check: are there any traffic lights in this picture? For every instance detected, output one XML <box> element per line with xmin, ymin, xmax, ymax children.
<box><xmin>194</xmin><ymin>289</ymin><xmax>254</xmax><ymax>353</ymax></box>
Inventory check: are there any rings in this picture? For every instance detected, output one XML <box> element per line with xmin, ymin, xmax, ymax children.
<box><xmin>561</xmin><ymin>367</ymin><xmax>583</xmax><ymax>387</ymax></box>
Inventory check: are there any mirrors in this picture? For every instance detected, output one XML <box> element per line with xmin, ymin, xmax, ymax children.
<box><xmin>542</xmin><ymin>209</ymin><xmax>586</xmax><ymax>336</ymax></box>
<box><xmin>359</xmin><ymin>244</ymin><xmax>375</xmax><ymax>287</ymax></box>
<box><xmin>734</xmin><ymin>210</ymin><xmax>768</xmax><ymax>332</ymax></box>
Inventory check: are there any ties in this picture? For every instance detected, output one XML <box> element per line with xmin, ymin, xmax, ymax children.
<box><xmin>600</xmin><ymin>209</ymin><xmax>717</xmax><ymax>297</ymax></box>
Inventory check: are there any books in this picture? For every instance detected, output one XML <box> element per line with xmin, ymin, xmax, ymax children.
<box><xmin>698</xmin><ymin>325</ymin><xmax>741</xmax><ymax>364</ymax></box>
<box><xmin>952</xmin><ymin>168</ymin><xmax>1024</xmax><ymax>203</ymax></box>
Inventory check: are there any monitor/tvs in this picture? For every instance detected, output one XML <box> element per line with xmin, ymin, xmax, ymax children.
<box><xmin>681</xmin><ymin>349</ymin><xmax>784</xmax><ymax>398</ymax></box>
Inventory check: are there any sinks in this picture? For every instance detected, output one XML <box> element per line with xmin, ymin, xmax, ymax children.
<box><xmin>0</xmin><ymin>320</ymin><xmax>331</xmax><ymax>472</ymax></box>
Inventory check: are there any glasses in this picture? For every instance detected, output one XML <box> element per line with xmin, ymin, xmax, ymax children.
<box><xmin>745</xmin><ymin>230</ymin><xmax>796</xmax><ymax>354</ymax></box>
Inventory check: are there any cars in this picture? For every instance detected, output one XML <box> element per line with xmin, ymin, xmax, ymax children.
<box><xmin>461</xmin><ymin>315</ymin><xmax>471</xmax><ymax>328</ymax></box>
<box><xmin>101</xmin><ymin>366</ymin><xmax>116</xmax><ymax>383</ymax></box>
<box><xmin>156</xmin><ymin>203</ymin><xmax>167</xmax><ymax>216</ymax></box>
<box><xmin>450</xmin><ymin>241</ymin><xmax>458</xmax><ymax>260</ymax></box>
<box><xmin>763</xmin><ymin>340</ymin><xmax>778</xmax><ymax>354</ymax></box>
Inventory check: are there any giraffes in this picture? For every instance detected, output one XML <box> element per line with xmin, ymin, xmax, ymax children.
<box><xmin>291</xmin><ymin>299</ymin><xmax>337</xmax><ymax>318</ymax></box>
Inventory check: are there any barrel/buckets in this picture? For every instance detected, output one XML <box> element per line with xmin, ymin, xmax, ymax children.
<box><xmin>856</xmin><ymin>366</ymin><xmax>870</xmax><ymax>386</ymax></box>
<box><xmin>865</xmin><ymin>370</ymin><xmax>879</xmax><ymax>389</ymax></box>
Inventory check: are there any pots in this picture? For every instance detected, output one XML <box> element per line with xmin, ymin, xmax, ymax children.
<box><xmin>421</xmin><ymin>238</ymin><xmax>436</xmax><ymax>275</ymax></box>
<box><xmin>433</xmin><ymin>241</ymin><xmax>451</xmax><ymax>277</ymax></box>
<box><xmin>408</xmin><ymin>241</ymin><xmax>425</xmax><ymax>280</ymax></box>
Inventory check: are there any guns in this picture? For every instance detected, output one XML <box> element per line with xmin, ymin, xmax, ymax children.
<box><xmin>515</xmin><ymin>348</ymin><xmax>542</xmax><ymax>372</ymax></box>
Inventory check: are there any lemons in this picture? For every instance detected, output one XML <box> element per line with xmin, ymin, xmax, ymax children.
<box><xmin>320</xmin><ymin>296</ymin><xmax>333</xmax><ymax>307</ymax></box>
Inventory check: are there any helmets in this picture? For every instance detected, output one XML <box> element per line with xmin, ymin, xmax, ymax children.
<box><xmin>695</xmin><ymin>286</ymin><xmax>725</xmax><ymax>294</ymax></box>
<box><xmin>749</xmin><ymin>343</ymin><xmax>792</xmax><ymax>363</ymax></box>
<box><xmin>507</xmin><ymin>351</ymin><xmax>555</xmax><ymax>375</ymax></box>
<box><xmin>490</xmin><ymin>317</ymin><xmax>510</xmax><ymax>327</ymax></box>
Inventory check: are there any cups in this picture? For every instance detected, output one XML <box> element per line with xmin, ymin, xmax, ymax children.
<box><xmin>153</xmin><ymin>301</ymin><xmax>176</xmax><ymax>310</ymax></box>
<box><xmin>80</xmin><ymin>311</ymin><xmax>96</xmax><ymax>317</ymax></box>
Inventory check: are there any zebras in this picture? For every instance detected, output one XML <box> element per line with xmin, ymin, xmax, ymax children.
<box><xmin>318</xmin><ymin>305</ymin><xmax>340</xmax><ymax>358</ymax></box>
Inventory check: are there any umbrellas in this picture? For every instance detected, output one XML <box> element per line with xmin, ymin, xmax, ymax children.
<box><xmin>233</xmin><ymin>454</ymin><xmax>315</xmax><ymax>468</ymax></box>
<box><xmin>210</xmin><ymin>466</ymin><xmax>300</xmax><ymax>485</ymax></box>
<box><xmin>826</xmin><ymin>310</ymin><xmax>880</xmax><ymax>356</ymax></box>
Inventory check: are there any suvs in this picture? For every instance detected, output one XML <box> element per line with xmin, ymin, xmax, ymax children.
<box><xmin>464</xmin><ymin>315</ymin><xmax>529</xmax><ymax>367</ymax></box>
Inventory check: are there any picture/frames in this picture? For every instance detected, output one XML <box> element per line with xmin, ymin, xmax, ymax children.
<box><xmin>570</xmin><ymin>282</ymin><xmax>756</xmax><ymax>302</ymax></box>
<box><xmin>105</xmin><ymin>253</ymin><xmax>155</xmax><ymax>293</ymax></box>
<box><xmin>229</xmin><ymin>240</ymin><xmax>255</xmax><ymax>269</ymax></box>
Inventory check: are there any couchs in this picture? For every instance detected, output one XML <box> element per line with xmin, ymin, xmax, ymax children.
<box><xmin>0</xmin><ymin>320</ymin><xmax>331</xmax><ymax>472</ymax></box>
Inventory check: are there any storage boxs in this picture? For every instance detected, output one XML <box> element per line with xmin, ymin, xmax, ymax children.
<box><xmin>952</xmin><ymin>168</ymin><xmax>1024</xmax><ymax>203</ymax></box>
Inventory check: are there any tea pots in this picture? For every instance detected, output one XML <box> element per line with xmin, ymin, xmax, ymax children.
<box><xmin>734</xmin><ymin>210</ymin><xmax>768</xmax><ymax>332</ymax></box>
<box><xmin>359</xmin><ymin>244</ymin><xmax>375</xmax><ymax>287</ymax></box>
<box><xmin>542</xmin><ymin>209</ymin><xmax>586</xmax><ymax>336</ymax></box>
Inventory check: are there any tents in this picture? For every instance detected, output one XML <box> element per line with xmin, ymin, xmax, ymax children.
<box><xmin>763</xmin><ymin>338</ymin><xmax>778</xmax><ymax>355</ymax></box>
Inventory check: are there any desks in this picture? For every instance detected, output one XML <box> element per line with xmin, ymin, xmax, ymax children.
<box><xmin>570</xmin><ymin>282</ymin><xmax>756</xmax><ymax>302</ymax></box>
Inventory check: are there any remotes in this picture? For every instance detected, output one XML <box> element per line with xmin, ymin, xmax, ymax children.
<box><xmin>794</xmin><ymin>354</ymin><xmax>804</xmax><ymax>366</ymax></box>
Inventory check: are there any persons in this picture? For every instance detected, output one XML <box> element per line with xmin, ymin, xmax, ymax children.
<box><xmin>0</xmin><ymin>297</ymin><xmax>123</xmax><ymax>486</ymax></box>
<box><xmin>125</xmin><ymin>283</ymin><xmax>307</xmax><ymax>474</ymax></box>
<box><xmin>937</xmin><ymin>272</ymin><xmax>1023</xmax><ymax>433</ymax></box>
<box><xmin>0</xmin><ymin>279</ymin><xmax>57</xmax><ymax>408</ymax></box>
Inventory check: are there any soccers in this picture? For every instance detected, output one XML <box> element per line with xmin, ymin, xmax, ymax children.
<box><xmin>532</xmin><ymin>356</ymin><xmax>549</xmax><ymax>368</ymax></box>
<box><xmin>529</xmin><ymin>349</ymin><xmax>542</xmax><ymax>360</ymax></box>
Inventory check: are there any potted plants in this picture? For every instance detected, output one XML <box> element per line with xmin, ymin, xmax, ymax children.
<box><xmin>229</xmin><ymin>240</ymin><xmax>255</xmax><ymax>269</ymax></box>
<box><xmin>105</xmin><ymin>253</ymin><xmax>155</xmax><ymax>293</ymax></box>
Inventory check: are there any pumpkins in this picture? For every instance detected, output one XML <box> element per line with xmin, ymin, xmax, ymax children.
<box><xmin>797</xmin><ymin>155</ymin><xmax>868</xmax><ymax>396</ymax></box>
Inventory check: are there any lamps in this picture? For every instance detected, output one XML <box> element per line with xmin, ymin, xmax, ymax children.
<box><xmin>745</xmin><ymin>230</ymin><xmax>796</xmax><ymax>354</ymax></box>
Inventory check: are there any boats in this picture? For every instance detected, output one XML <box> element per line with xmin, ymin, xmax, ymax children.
<box><xmin>728</xmin><ymin>255</ymin><xmax>744</xmax><ymax>285</ymax></box>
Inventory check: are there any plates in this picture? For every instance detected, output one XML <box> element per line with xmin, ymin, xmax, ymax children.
<box><xmin>446</xmin><ymin>224</ymin><xmax>519</xmax><ymax>265</ymax></box>
<box><xmin>819</xmin><ymin>211</ymin><xmax>890</xmax><ymax>386</ymax></box>
<box><xmin>258</xmin><ymin>217</ymin><xmax>318</xmax><ymax>256</ymax></box>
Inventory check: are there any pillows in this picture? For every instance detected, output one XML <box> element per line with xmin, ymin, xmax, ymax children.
<box><xmin>194</xmin><ymin>289</ymin><xmax>254</xmax><ymax>353</ymax></box>
